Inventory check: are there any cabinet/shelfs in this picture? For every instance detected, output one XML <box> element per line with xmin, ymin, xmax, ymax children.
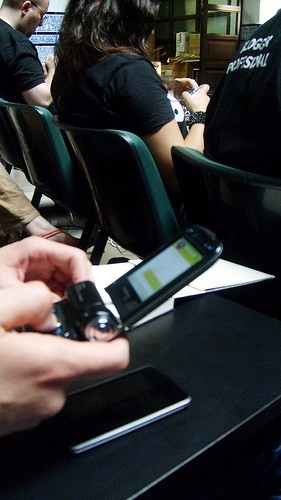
<box><xmin>142</xmin><ymin>0</ymin><xmax>243</xmax><ymax>92</ymax></box>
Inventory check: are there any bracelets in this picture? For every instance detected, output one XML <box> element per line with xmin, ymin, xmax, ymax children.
<box><xmin>189</xmin><ymin>111</ymin><xmax>206</xmax><ymax>130</ymax></box>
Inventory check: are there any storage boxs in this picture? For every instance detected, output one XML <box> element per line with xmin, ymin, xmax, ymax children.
<box><xmin>150</xmin><ymin>34</ymin><xmax>201</xmax><ymax>79</ymax></box>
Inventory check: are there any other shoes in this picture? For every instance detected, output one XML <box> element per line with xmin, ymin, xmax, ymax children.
<box><xmin>41</xmin><ymin>228</ymin><xmax>86</xmax><ymax>252</ymax></box>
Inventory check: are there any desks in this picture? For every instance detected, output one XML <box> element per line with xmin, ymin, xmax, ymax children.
<box><xmin>0</xmin><ymin>293</ymin><xmax>281</xmax><ymax>500</ymax></box>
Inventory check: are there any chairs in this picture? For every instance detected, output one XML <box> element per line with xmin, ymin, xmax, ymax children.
<box><xmin>0</xmin><ymin>98</ymin><xmax>281</xmax><ymax>266</ymax></box>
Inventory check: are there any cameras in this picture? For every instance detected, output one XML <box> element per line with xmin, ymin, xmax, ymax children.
<box><xmin>176</xmin><ymin>87</ymin><xmax>198</xmax><ymax>105</ymax></box>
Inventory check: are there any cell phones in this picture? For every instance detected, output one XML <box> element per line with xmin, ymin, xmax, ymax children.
<box><xmin>11</xmin><ymin>224</ymin><xmax>225</xmax><ymax>343</ymax></box>
<box><xmin>55</xmin><ymin>366</ymin><xmax>194</xmax><ymax>453</ymax></box>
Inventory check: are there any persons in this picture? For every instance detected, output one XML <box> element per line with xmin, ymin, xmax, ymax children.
<box><xmin>51</xmin><ymin>0</ymin><xmax>213</xmax><ymax>229</ymax></box>
<box><xmin>0</xmin><ymin>161</ymin><xmax>98</xmax><ymax>251</ymax></box>
<box><xmin>204</xmin><ymin>8</ymin><xmax>281</xmax><ymax>179</ymax></box>
<box><xmin>0</xmin><ymin>0</ymin><xmax>58</xmax><ymax>123</ymax></box>
<box><xmin>0</xmin><ymin>235</ymin><xmax>131</xmax><ymax>437</ymax></box>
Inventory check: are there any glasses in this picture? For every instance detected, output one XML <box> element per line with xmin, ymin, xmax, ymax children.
<box><xmin>30</xmin><ymin>0</ymin><xmax>48</xmax><ymax>21</ymax></box>
